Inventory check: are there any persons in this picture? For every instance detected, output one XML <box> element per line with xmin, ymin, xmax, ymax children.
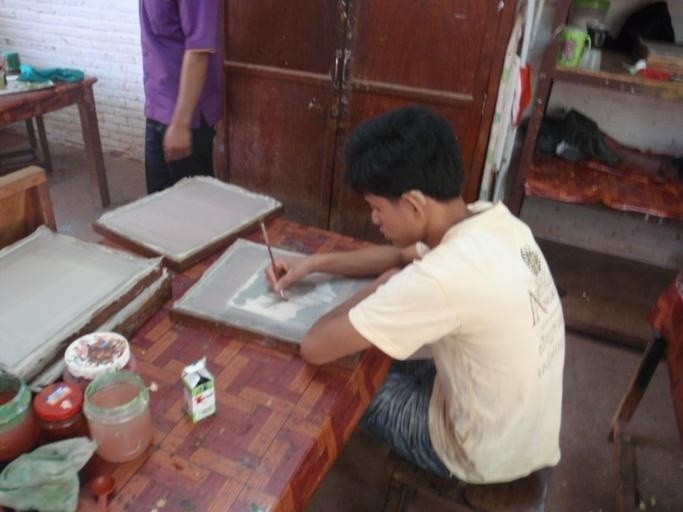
<box><xmin>263</xmin><ymin>103</ymin><xmax>567</xmax><ymax>489</ymax></box>
<box><xmin>135</xmin><ymin>1</ymin><xmax>224</xmax><ymax>194</ymax></box>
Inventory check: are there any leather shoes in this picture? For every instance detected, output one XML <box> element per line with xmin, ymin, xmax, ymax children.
<box><xmin>557</xmin><ymin>110</ymin><xmax>620</xmax><ymax>166</ymax></box>
<box><xmin>521</xmin><ymin>116</ymin><xmax>557</xmax><ymax>152</ymax></box>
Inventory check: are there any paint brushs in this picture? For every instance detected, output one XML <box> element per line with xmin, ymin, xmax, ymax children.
<box><xmin>258</xmin><ymin>219</ymin><xmax>285</xmax><ymax>300</ymax></box>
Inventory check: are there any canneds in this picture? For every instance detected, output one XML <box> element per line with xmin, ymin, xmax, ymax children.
<box><xmin>32</xmin><ymin>382</ymin><xmax>88</xmax><ymax>443</ymax></box>
<box><xmin>63</xmin><ymin>331</ymin><xmax>137</xmax><ymax>392</ymax></box>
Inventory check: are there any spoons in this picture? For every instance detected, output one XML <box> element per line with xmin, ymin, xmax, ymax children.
<box><xmin>92</xmin><ymin>474</ymin><xmax>116</xmax><ymax>511</ymax></box>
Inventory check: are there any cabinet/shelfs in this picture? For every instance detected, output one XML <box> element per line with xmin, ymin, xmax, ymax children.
<box><xmin>507</xmin><ymin>0</ymin><xmax>683</xmax><ymax>362</ymax></box>
<box><xmin>202</xmin><ymin>0</ymin><xmax>521</xmax><ymax>245</ymax></box>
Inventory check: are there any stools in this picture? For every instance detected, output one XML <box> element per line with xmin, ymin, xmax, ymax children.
<box><xmin>384</xmin><ymin>440</ymin><xmax>550</xmax><ymax>509</ymax></box>
<box><xmin>0</xmin><ymin>112</ymin><xmax>54</xmax><ymax>175</ymax></box>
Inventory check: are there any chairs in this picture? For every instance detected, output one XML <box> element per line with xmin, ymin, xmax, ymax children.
<box><xmin>0</xmin><ymin>163</ymin><xmax>58</xmax><ymax>249</ymax></box>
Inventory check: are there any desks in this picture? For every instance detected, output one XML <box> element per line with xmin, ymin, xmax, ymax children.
<box><xmin>0</xmin><ymin>193</ymin><xmax>420</xmax><ymax>512</ymax></box>
<box><xmin>0</xmin><ymin>72</ymin><xmax>111</xmax><ymax>209</ymax></box>
<box><xmin>601</xmin><ymin>270</ymin><xmax>681</xmax><ymax>511</ymax></box>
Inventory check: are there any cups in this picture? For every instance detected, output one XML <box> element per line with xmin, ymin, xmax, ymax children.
<box><xmin>580</xmin><ymin>49</ymin><xmax>602</xmax><ymax>71</ymax></box>
<box><xmin>555</xmin><ymin>25</ymin><xmax>592</xmax><ymax>66</ymax></box>
